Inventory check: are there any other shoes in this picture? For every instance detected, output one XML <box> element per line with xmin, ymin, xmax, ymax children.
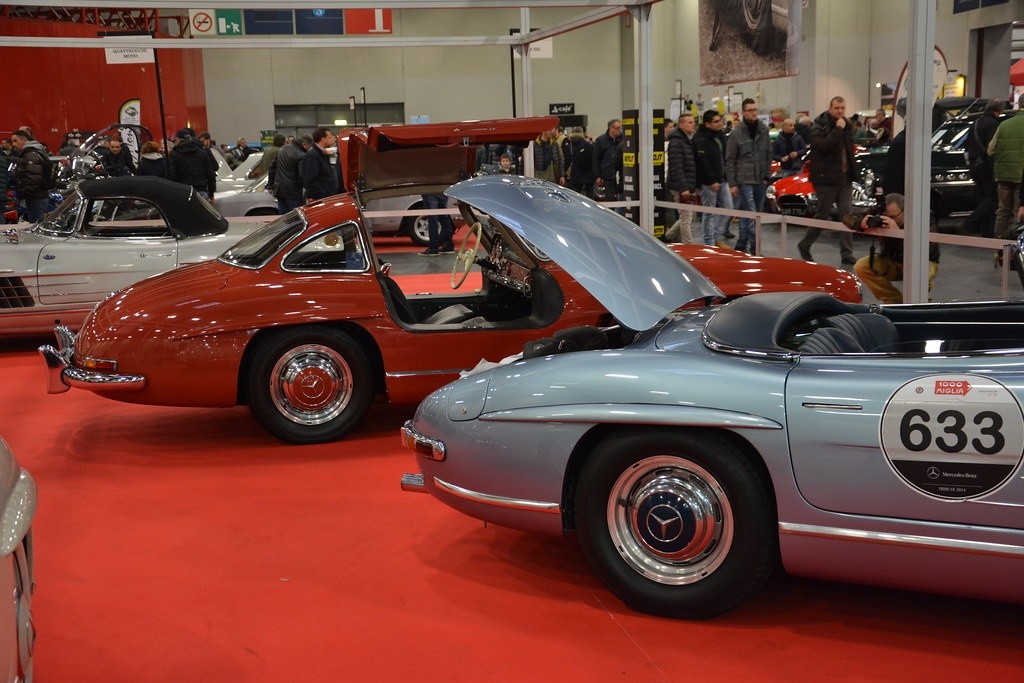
<box><xmin>715</xmin><ymin>241</ymin><xmax>733</xmax><ymax>249</ymax></box>
<box><xmin>797</xmin><ymin>242</ymin><xmax>813</xmax><ymax>262</ymax></box>
<box><xmin>841</xmin><ymin>256</ymin><xmax>858</xmax><ymax>265</ymax></box>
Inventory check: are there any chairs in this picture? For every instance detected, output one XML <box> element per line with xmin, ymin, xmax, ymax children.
<box><xmin>378</xmin><ymin>256</ymin><xmax>490</xmax><ymax>325</ymax></box>
<box><xmin>798</xmin><ymin>313</ymin><xmax>876</xmax><ymax>354</ymax></box>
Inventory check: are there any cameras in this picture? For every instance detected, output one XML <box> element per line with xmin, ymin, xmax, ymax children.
<box><xmin>866</xmin><ymin>185</ymin><xmax>887</xmax><ymax>227</ymax></box>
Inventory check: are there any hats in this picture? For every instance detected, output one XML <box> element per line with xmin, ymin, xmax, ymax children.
<box><xmin>176</xmin><ymin>129</ymin><xmax>191</xmax><ymax>139</ymax></box>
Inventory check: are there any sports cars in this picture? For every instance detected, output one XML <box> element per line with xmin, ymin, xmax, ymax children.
<box><xmin>0</xmin><ymin>124</ymin><xmax>469</xmax><ymax>340</ymax></box>
<box><xmin>763</xmin><ymin>98</ymin><xmax>1018</xmax><ymax>235</ymax></box>
<box><xmin>397</xmin><ymin>172</ymin><xmax>1023</xmax><ymax>617</ymax></box>
<box><xmin>36</xmin><ymin>114</ymin><xmax>862</xmax><ymax>447</ymax></box>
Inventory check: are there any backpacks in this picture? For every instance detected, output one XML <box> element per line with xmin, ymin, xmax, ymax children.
<box><xmin>29</xmin><ymin>149</ymin><xmax>59</xmax><ymax>191</ymax></box>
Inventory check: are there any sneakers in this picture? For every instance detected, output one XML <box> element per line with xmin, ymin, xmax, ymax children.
<box><xmin>439</xmin><ymin>244</ymin><xmax>455</xmax><ymax>253</ymax></box>
<box><xmin>418</xmin><ymin>248</ymin><xmax>439</xmax><ymax>256</ymax></box>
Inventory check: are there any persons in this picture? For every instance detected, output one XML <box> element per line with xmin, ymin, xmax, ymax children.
<box><xmin>843</xmin><ymin>193</ymin><xmax>940</xmax><ymax>304</ymax></box>
<box><xmin>881</xmin><ymin>98</ymin><xmax>906</xmax><ymax>197</ymax></box>
<box><xmin>134</xmin><ymin>141</ymin><xmax>167</xmax><ymax>178</ymax></box>
<box><xmin>166</xmin><ymin>128</ymin><xmax>219</xmax><ymax>207</ymax></box>
<box><xmin>474</xmin><ymin>125</ymin><xmax>598</xmax><ymax>202</ymax></box>
<box><xmin>95</xmin><ymin>136</ymin><xmax>137</xmax><ymax>180</ymax></box>
<box><xmin>967</xmin><ymin>93</ymin><xmax>1024</xmax><ymax>290</ymax></box>
<box><xmin>167</xmin><ymin>131</ymin><xmax>259</xmax><ymax>171</ymax></box>
<box><xmin>593</xmin><ymin>119</ymin><xmax>623</xmax><ymax>216</ymax></box>
<box><xmin>11</xmin><ymin>130</ymin><xmax>50</xmax><ymax>224</ymax></box>
<box><xmin>0</xmin><ymin>126</ymin><xmax>49</xmax><ymax>224</ymax></box>
<box><xmin>303</xmin><ymin>127</ymin><xmax>338</xmax><ymax>200</ymax></box>
<box><xmin>496</xmin><ymin>153</ymin><xmax>516</xmax><ymax>176</ymax></box>
<box><xmin>614</xmin><ymin>141</ymin><xmax>623</xmax><ymax>217</ymax></box>
<box><xmin>268</xmin><ymin>133</ymin><xmax>314</xmax><ymax>215</ymax></box>
<box><xmin>797</xmin><ymin>96</ymin><xmax>859</xmax><ymax>264</ymax></box>
<box><xmin>417</xmin><ymin>193</ymin><xmax>455</xmax><ymax>257</ymax></box>
<box><xmin>57</xmin><ymin>141</ymin><xmax>84</xmax><ymax>156</ymax></box>
<box><xmin>261</xmin><ymin>134</ymin><xmax>286</xmax><ymax>177</ymax></box>
<box><xmin>665</xmin><ymin>99</ymin><xmax>892</xmax><ymax>259</ymax></box>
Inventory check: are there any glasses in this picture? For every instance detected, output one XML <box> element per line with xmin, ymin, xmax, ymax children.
<box><xmin>888</xmin><ymin>210</ymin><xmax>902</xmax><ymax>219</ymax></box>
<box><xmin>744</xmin><ymin>108</ymin><xmax>757</xmax><ymax>112</ymax></box>
